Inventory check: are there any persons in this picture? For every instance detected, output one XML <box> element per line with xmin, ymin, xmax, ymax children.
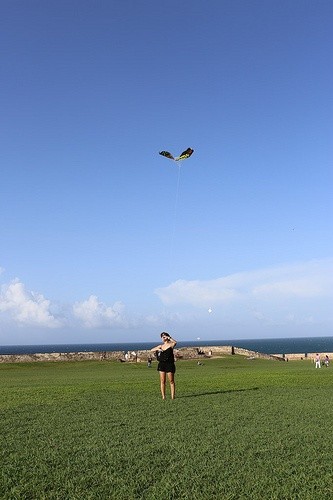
<box><xmin>150</xmin><ymin>332</ymin><xmax>177</xmax><ymax>399</ymax></box>
<box><xmin>99</xmin><ymin>354</ymin><xmax>104</xmax><ymax>364</ymax></box>
<box><xmin>315</xmin><ymin>354</ymin><xmax>321</xmax><ymax>368</ymax></box>
<box><xmin>324</xmin><ymin>355</ymin><xmax>329</xmax><ymax>367</ymax></box>
<box><xmin>148</xmin><ymin>354</ymin><xmax>152</xmax><ymax>368</ymax></box>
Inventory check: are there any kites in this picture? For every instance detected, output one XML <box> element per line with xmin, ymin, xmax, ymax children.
<box><xmin>158</xmin><ymin>147</ymin><xmax>194</xmax><ymax>161</ymax></box>
